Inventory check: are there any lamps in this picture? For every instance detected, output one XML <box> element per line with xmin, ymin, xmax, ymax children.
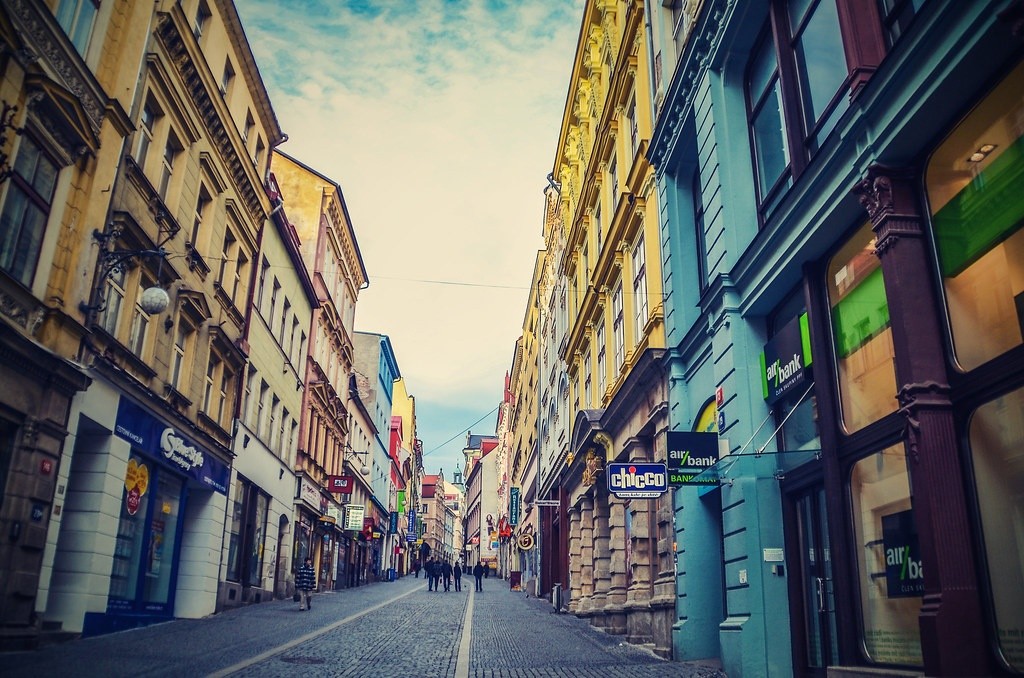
<box><xmin>78</xmin><ymin>227</ymin><xmax>173</xmax><ymax>321</ymax></box>
<box><xmin>413</xmin><ymin>505</ymin><xmax>425</xmax><ymax>523</ymax></box>
<box><xmin>341</xmin><ymin>450</ymin><xmax>370</xmax><ymax>475</ymax></box>
<box><xmin>389</xmin><ymin>488</ymin><xmax>407</xmax><ymax>507</ymax></box>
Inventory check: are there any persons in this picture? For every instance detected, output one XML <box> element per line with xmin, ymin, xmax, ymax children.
<box><xmin>295</xmin><ymin>558</ymin><xmax>316</xmax><ymax>611</ymax></box>
<box><xmin>413</xmin><ymin>560</ymin><xmax>421</xmax><ymax>578</ymax></box>
<box><xmin>426</xmin><ymin>556</ymin><xmax>435</xmax><ymax>591</ymax></box>
<box><xmin>473</xmin><ymin>561</ymin><xmax>483</xmax><ymax>592</ymax></box>
<box><xmin>424</xmin><ymin>558</ymin><xmax>429</xmax><ymax>579</ymax></box>
<box><xmin>453</xmin><ymin>562</ymin><xmax>462</xmax><ymax>591</ymax></box>
<box><xmin>483</xmin><ymin>562</ymin><xmax>490</xmax><ymax>578</ymax></box>
<box><xmin>432</xmin><ymin>560</ymin><xmax>442</xmax><ymax>591</ymax></box>
<box><xmin>441</xmin><ymin>560</ymin><xmax>453</xmax><ymax>591</ymax></box>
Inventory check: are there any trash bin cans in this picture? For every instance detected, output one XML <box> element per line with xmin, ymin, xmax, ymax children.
<box><xmin>387</xmin><ymin>568</ymin><xmax>394</xmax><ymax>582</ymax></box>
<box><xmin>467</xmin><ymin>566</ymin><xmax>472</xmax><ymax>575</ymax></box>
<box><xmin>509</xmin><ymin>570</ymin><xmax>522</xmax><ymax>592</ymax></box>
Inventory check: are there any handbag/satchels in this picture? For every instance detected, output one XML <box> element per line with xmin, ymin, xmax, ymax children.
<box><xmin>293</xmin><ymin>591</ymin><xmax>301</xmax><ymax>601</ymax></box>
